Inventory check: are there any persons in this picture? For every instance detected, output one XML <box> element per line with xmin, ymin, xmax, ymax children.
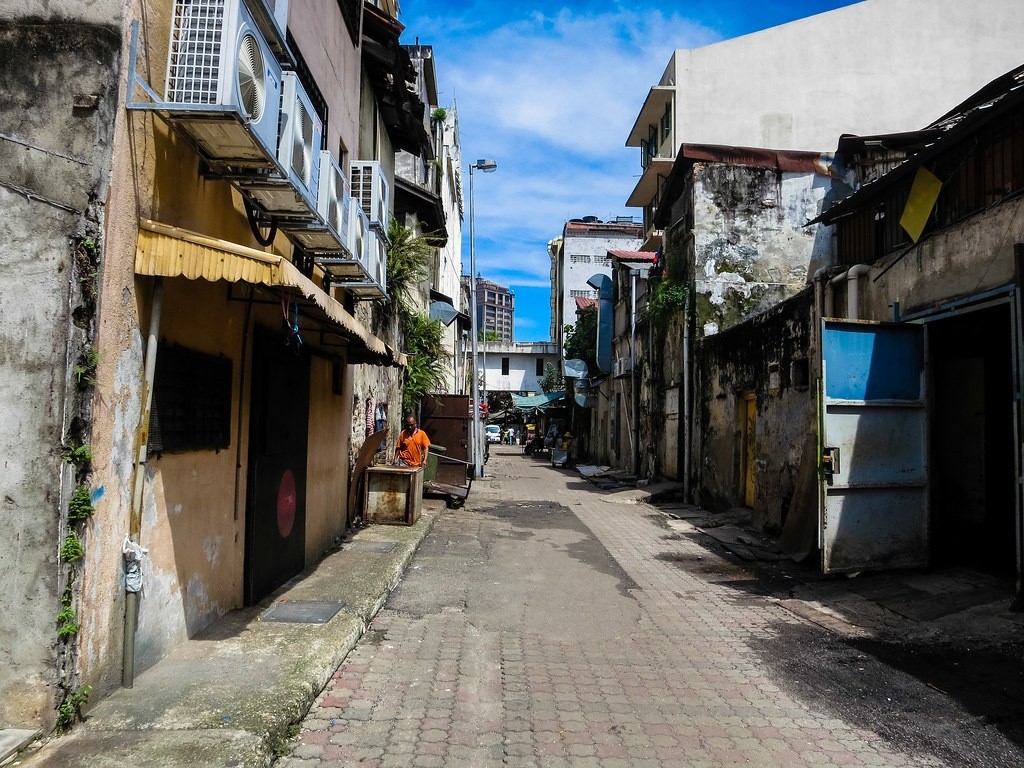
<box><xmin>555</xmin><ymin>435</ymin><xmax>563</xmax><ymax>448</ymax></box>
<box><xmin>499</xmin><ymin>429</ymin><xmax>505</xmax><ymax>445</ymax></box>
<box><xmin>393</xmin><ymin>415</ymin><xmax>431</xmax><ymax>468</ymax></box>
<box><xmin>504</xmin><ymin>426</ymin><xmax>514</xmax><ymax>445</ymax></box>
<box><xmin>563</xmin><ymin>432</ymin><xmax>573</xmax><ymax>466</ymax></box>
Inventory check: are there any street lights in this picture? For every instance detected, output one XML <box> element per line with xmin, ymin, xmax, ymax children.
<box><xmin>468</xmin><ymin>158</ymin><xmax>497</xmax><ymax>482</ymax></box>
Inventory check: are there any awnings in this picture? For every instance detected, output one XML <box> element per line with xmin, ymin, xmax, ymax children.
<box><xmin>134</xmin><ymin>217</ymin><xmax>408</xmax><ymax>367</ymax></box>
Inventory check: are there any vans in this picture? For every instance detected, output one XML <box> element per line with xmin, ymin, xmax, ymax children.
<box><xmin>485</xmin><ymin>424</ymin><xmax>500</xmax><ymax>443</ymax></box>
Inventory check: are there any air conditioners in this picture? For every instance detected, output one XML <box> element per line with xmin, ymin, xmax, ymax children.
<box><xmin>617</xmin><ymin>357</ymin><xmax>631</xmax><ymax>377</ymax></box>
<box><xmin>164</xmin><ymin>0</ymin><xmax>394</xmax><ymax>306</ymax></box>
